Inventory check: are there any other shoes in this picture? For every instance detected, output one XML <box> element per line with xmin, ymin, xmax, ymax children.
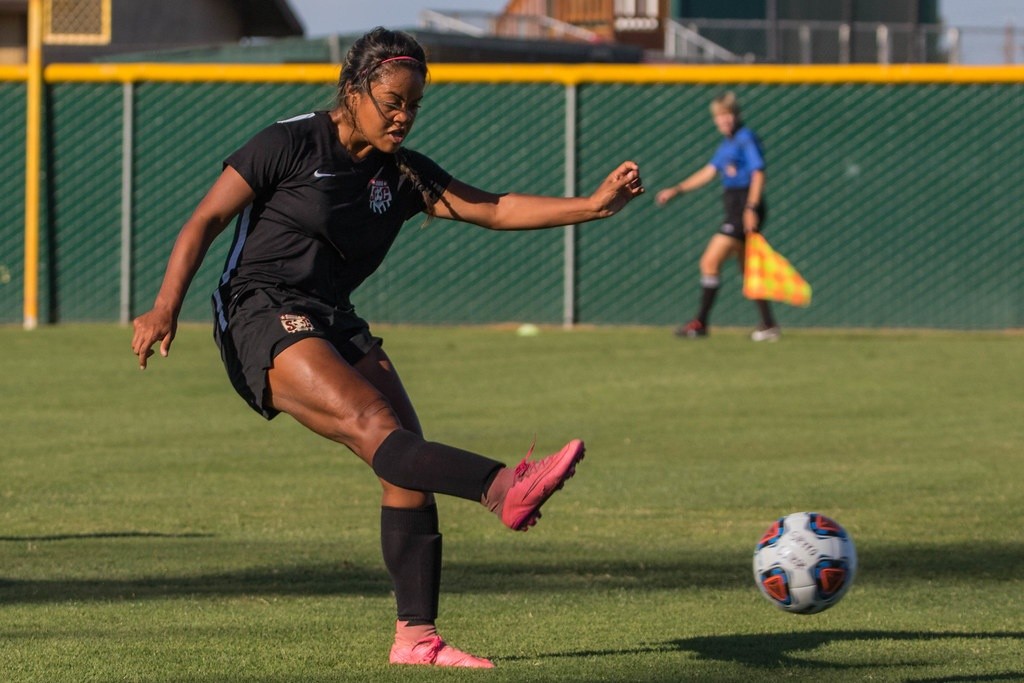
<box><xmin>747</xmin><ymin>327</ymin><xmax>782</xmax><ymax>343</ymax></box>
<box><xmin>676</xmin><ymin>321</ymin><xmax>708</xmax><ymax>338</ymax></box>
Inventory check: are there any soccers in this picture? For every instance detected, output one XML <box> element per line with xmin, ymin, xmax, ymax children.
<box><xmin>753</xmin><ymin>510</ymin><xmax>859</xmax><ymax>618</ymax></box>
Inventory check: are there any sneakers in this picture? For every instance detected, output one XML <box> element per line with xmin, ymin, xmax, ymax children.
<box><xmin>389</xmin><ymin>633</ymin><xmax>495</xmax><ymax>669</ymax></box>
<box><xmin>500</xmin><ymin>432</ymin><xmax>586</xmax><ymax>531</ymax></box>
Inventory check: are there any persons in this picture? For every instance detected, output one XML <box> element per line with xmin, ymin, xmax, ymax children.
<box><xmin>128</xmin><ymin>26</ymin><xmax>646</xmax><ymax>670</ymax></box>
<box><xmin>653</xmin><ymin>92</ymin><xmax>784</xmax><ymax>343</ymax></box>
